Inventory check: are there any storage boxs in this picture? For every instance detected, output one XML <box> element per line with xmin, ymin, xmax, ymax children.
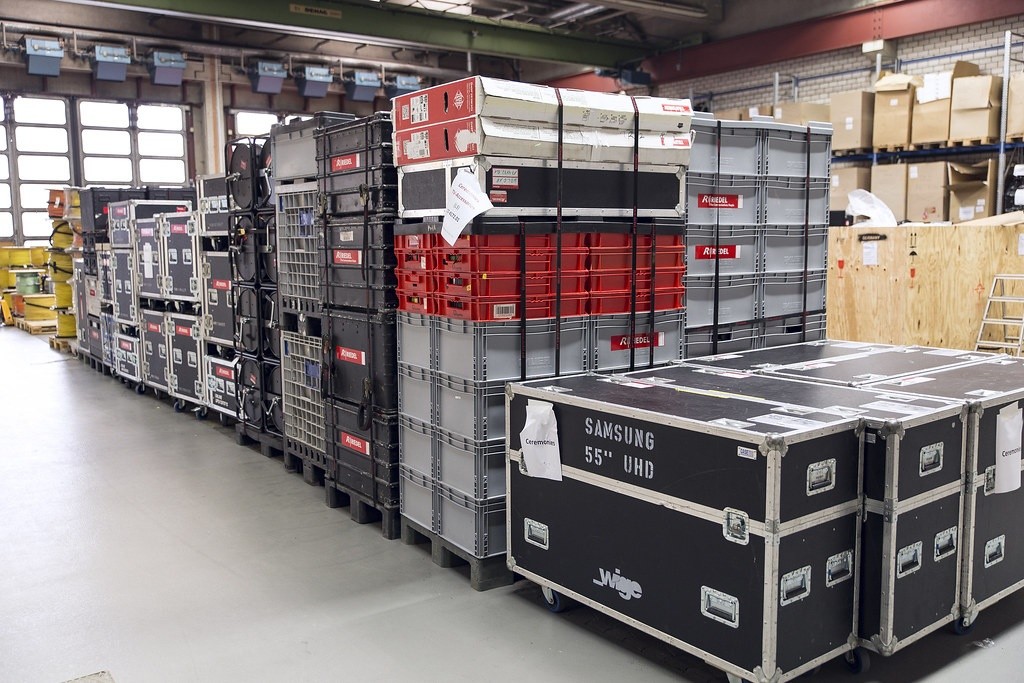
<box><xmin>0</xmin><ymin>61</ymin><xmax>1024</xmax><ymax>683</ymax></box>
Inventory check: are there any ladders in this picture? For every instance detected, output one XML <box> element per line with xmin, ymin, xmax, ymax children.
<box><xmin>974</xmin><ymin>273</ymin><xmax>1024</xmax><ymax>357</ymax></box>
